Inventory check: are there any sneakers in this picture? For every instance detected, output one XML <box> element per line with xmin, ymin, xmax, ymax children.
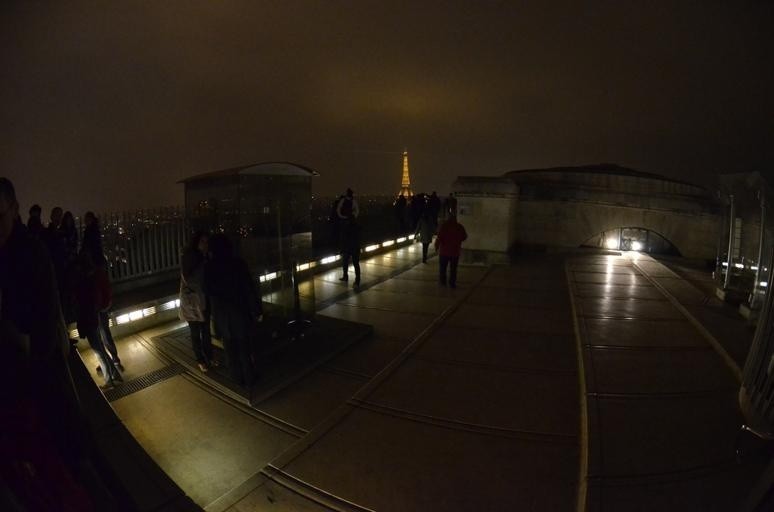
<box><xmin>94</xmin><ymin>357</ymin><xmax>122</xmax><ymax>390</ymax></box>
<box><xmin>197</xmin><ymin>355</ymin><xmax>219</xmax><ymax>372</ymax></box>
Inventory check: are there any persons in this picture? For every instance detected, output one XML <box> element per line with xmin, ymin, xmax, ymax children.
<box><xmin>0</xmin><ymin>177</ymin><xmax>123</xmax><ymax>389</ymax></box>
<box><xmin>178</xmin><ymin>226</ymin><xmax>268</xmax><ymax>387</ymax></box>
<box><xmin>395</xmin><ymin>190</ymin><xmax>457</xmax><ymax>264</ymax></box>
<box><xmin>434</xmin><ymin>213</ymin><xmax>467</xmax><ymax>290</ymax></box>
<box><xmin>331</xmin><ymin>187</ymin><xmax>362</xmax><ymax>286</ymax></box>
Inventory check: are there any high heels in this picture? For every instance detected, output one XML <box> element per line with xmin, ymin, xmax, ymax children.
<box><xmin>340</xmin><ymin>276</ymin><xmax>360</xmax><ymax>285</ymax></box>
